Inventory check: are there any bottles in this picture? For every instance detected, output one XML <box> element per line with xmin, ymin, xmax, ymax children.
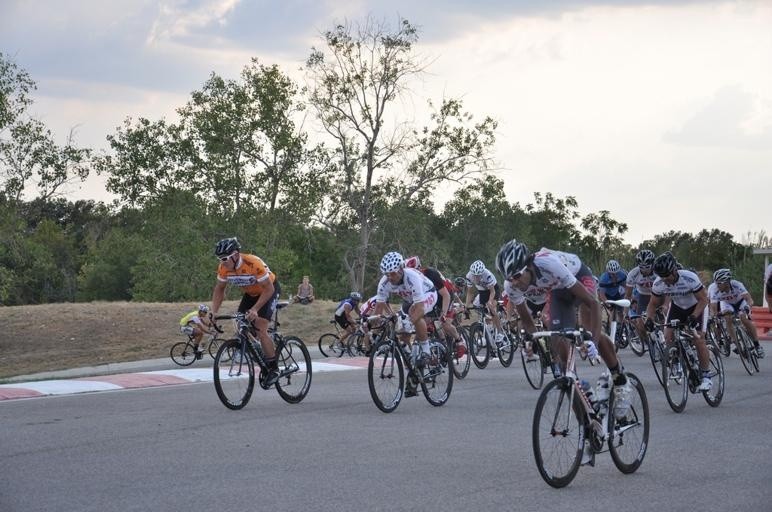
<box><xmin>581</xmin><ymin>379</ymin><xmax>598</xmax><ymax>404</ymax></box>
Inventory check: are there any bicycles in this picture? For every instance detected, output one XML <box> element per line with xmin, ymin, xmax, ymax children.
<box><xmin>522</xmin><ymin>298</ymin><xmax>767</xmax><ymax>391</ymax></box>
<box><xmin>656</xmin><ymin>320</ymin><xmax>725</xmax><ymax>413</ymax></box>
<box><xmin>521</xmin><ymin>329</ymin><xmax>650</xmax><ymax>489</ymax></box>
<box><xmin>210</xmin><ymin>309</ymin><xmax>313</xmax><ymax>410</ymax></box>
<box><xmin>361</xmin><ymin>311</ymin><xmax>453</xmax><ymax>413</ymax></box>
<box><xmin>318</xmin><ymin>305</ymin><xmax>516</xmax><ymax>379</ymax></box>
<box><xmin>171</xmin><ymin>326</ymin><xmax>235</xmax><ymax>366</ymax></box>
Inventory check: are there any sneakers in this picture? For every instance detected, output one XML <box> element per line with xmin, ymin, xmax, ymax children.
<box><xmin>335</xmin><ymin>339</ymin><xmax>344</xmax><ymax>350</ymax></box>
<box><xmin>194</xmin><ymin>349</ymin><xmax>205</xmax><ymax>353</ymax></box>
<box><xmin>405</xmin><ymin>372</ymin><xmax>417</xmax><ymax>398</ymax></box>
<box><xmin>261</xmin><ymin>369</ymin><xmax>281</xmax><ymax>390</ymax></box>
<box><xmin>579</xmin><ymin>440</ymin><xmax>592</xmax><ymax>465</ymax></box>
<box><xmin>456</xmin><ymin>336</ymin><xmax>466</xmax><ymax>358</ymax></box>
<box><xmin>757</xmin><ymin>346</ymin><xmax>764</xmax><ymax>358</ymax></box>
<box><xmin>496</xmin><ymin>329</ymin><xmax>506</xmax><ymax>342</ymax></box>
<box><xmin>613</xmin><ymin>374</ymin><xmax>632</xmax><ymax>419</ymax></box>
<box><xmin>699</xmin><ymin>378</ymin><xmax>713</xmax><ymax>390</ymax></box>
<box><xmin>417</xmin><ymin>350</ymin><xmax>434</xmax><ymax>368</ymax></box>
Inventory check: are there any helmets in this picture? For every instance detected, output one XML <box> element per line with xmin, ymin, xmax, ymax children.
<box><xmin>495</xmin><ymin>238</ymin><xmax>530</xmax><ymax>280</ymax></box>
<box><xmin>350</xmin><ymin>292</ymin><xmax>363</xmax><ymax>300</ymax></box>
<box><xmin>456</xmin><ymin>277</ymin><xmax>467</xmax><ymax>288</ymax></box>
<box><xmin>469</xmin><ymin>260</ymin><xmax>485</xmax><ymax>275</ymax></box>
<box><xmin>607</xmin><ymin>250</ymin><xmax>731</xmax><ymax>282</ymax></box>
<box><xmin>199</xmin><ymin>304</ymin><xmax>209</xmax><ymax>311</ymax></box>
<box><xmin>215</xmin><ymin>238</ymin><xmax>241</xmax><ymax>257</ymax></box>
<box><xmin>405</xmin><ymin>256</ymin><xmax>422</xmax><ymax>270</ymax></box>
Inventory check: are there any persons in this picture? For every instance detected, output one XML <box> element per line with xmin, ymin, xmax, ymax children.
<box><xmin>450</xmin><ymin>276</ymin><xmax>467</xmax><ymax>315</ymax></box>
<box><xmin>180</xmin><ymin>304</ymin><xmax>219</xmax><ymax>355</ymax></box>
<box><xmin>206</xmin><ymin>237</ymin><xmax>283</xmax><ymax>386</ymax></box>
<box><xmin>494</xmin><ymin>238</ymin><xmax>637</xmax><ymax>465</ymax></box>
<box><xmin>292</xmin><ymin>275</ymin><xmax>314</xmax><ymax>305</ymax></box>
<box><xmin>465</xmin><ymin>259</ymin><xmax>506</xmax><ymax>343</ymax></box>
<box><xmin>623</xmin><ymin>249</ymin><xmax>658</xmax><ymax>345</ymax></box>
<box><xmin>360</xmin><ymin>250</ymin><xmax>442</xmax><ymax>399</ymax></box>
<box><xmin>333</xmin><ymin>291</ymin><xmax>363</xmax><ymax>345</ymax></box>
<box><xmin>597</xmin><ymin>259</ymin><xmax>637</xmax><ymax>349</ymax></box>
<box><xmin>645</xmin><ymin>251</ymin><xmax>713</xmax><ymax>391</ymax></box>
<box><xmin>706</xmin><ymin>268</ymin><xmax>766</xmax><ymax>360</ymax></box>
<box><xmin>401</xmin><ymin>255</ymin><xmax>467</xmax><ymax>362</ymax></box>
<box><xmin>501</xmin><ymin>290</ymin><xmax>516</xmax><ymax>320</ymax></box>
<box><xmin>518</xmin><ymin>287</ymin><xmax>553</xmax><ymax>374</ymax></box>
<box><xmin>359</xmin><ymin>293</ymin><xmax>395</xmax><ymax>356</ymax></box>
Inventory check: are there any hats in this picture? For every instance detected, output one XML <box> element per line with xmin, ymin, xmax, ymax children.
<box><xmin>380</xmin><ymin>252</ymin><xmax>403</xmax><ymax>274</ymax></box>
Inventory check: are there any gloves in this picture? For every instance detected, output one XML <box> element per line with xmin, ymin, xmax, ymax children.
<box><xmin>404</xmin><ymin>320</ymin><xmax>413</xmax><ymax>332</ymax></box>
<box><xmin>686</xmin><ymin>315</ymin><xmax>699</xmax><ymax>328</ymax></box>
<box><xmin>644</xmin><ymin>317</ymin><xmax>655</xmax><ymax>333</ymax></box>
<box><xmin>584</xmin><ymin>337</ymin><xmax>598</xmax><ymax>360</ymax></box>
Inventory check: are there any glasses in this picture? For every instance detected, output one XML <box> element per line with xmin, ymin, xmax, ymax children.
<box><xmin>507</xmin><ymin>264</ymin><xmax>527</xmax><ymax>282</ymax></box>
<box><xmin>202</xmin><ymin>311</ymin><xmax>208</xmax><ymax>313</ymax></box>
<box><xmin>637</xmin><ymin>264</ymin><xmax>652</xmax><ymax>269</ymax></box>
<box><xmin>215</xmin><ymin>252</ymin><xmax>237</xmax><ymax>262</ymax></box>
